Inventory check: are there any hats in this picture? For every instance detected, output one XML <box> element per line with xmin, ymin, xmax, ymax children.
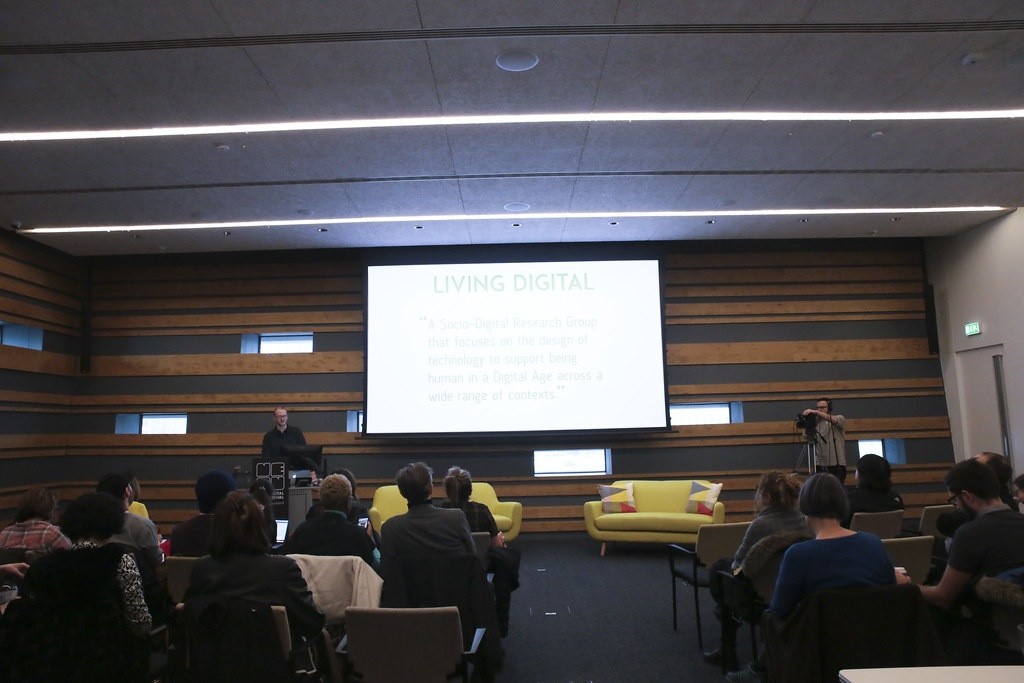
<box><xmin>196</xmin><ymin>469</ymin><xmax>236</xmax><ymax>514</ymax></box>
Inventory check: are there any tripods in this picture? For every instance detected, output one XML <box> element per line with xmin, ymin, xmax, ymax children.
<box><xmin>793</xmin><ymin>428</ymin><xmax>828</xmax><ymax>477</ymax></box>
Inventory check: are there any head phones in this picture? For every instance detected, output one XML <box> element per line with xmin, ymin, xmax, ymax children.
<box><xmin>823</xmin><ymin>398</ymin><xmax>833</xmax><ymax>413</ymax></box>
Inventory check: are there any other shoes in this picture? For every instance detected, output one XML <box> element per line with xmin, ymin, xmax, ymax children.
<box><xmin>703</xmin><ymin>648</ymin><xmax>738</xmax><ymax>665</ymax></box>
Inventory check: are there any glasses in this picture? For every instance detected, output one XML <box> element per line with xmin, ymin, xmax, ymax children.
<box><xmin>817</xmin><ymin>406</ymin><xmax>826</xmax><ymax>410</ymax></box>
<box><xmin>948</xmin><ymin>493</ymin><xmax>958</xmax><ymax>506</ymax></box>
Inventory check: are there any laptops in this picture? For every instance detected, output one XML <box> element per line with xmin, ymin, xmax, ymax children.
<box><xmin>272</xmin><ymin>519</ymin><xmax>289</xmax><ymax>549</ymax></box>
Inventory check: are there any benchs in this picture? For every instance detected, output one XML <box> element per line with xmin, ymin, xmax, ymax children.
<box><xmin>584</xmin><ymin>478</ymin><xmax>725</xmax><ymax>558</ymax></box>
<box><xmin>368</xmin><ymin>482</ymin><xmax>524</xmax><ymax>541</ymax></box>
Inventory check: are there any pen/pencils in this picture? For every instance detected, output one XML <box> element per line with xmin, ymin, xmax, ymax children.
<box><xmin>158</xmin><ymin>528</ymin><xmax>161</xmax><ymax>534</ymax></box>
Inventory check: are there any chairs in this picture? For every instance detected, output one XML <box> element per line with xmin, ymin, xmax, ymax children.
<box><xmin>142</xmin><ymin>531</ymin><xmax>502</xmax><ymax>683</ymax></box>
<box><xmin>666</xmin><ymin>501</ymin><xmax>960</xmax><ymax>683</ymax></box>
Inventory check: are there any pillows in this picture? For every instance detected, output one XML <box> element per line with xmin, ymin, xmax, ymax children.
<box><xmin>684</xmin><ymin>480</ymin><xmax>724</xmax><ymax>517</ymax></box>
<box><xmin>596</xmin><ymin>482</ymin><xmax>637</xmax><ymax>514</ymax></box>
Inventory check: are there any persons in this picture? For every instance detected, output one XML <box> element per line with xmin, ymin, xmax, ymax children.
<box><xmin>804</xmin><ymin>398</ymin><xmax>847</xmax><ymax>488</ymax></box>
<box><xmin>262</xmin><ymin>405</ymin><xmax>318</xmax><ymax>486</ymax></box>
<box><xmin>284</xmin><ymin>473</ymin><xmax>376</xmax><ymax>565</ymax></box>
<box><xmin>377</xmin><ymin>462</ymin><xmax>505</xmax><ymax>675</ymax></box>
<box><xmin>170</xmin><ymin>470</ymin><xmax>327</xmax><ymax>683</ymax></box>
<box><xmin>840</xmin><ymin>454</ymin><xmax>905</xmax><ymax>529</ymax></box>
<box><xmin>724</xmin><ymin>473</ymin><xmax>898</xmax><ymax>683</ymax></box>
<box><xmin>0</xmin><ymin>470</ymin><xmax>180</xmax><ymax>683</ymax></box>
<box><xmin>702</xmin><ymin>468</ymin><xmax>817</xmax><ymax>669</ymax></box>
<box><xmin>306</xmin><ymin>469</ymin><xmax>381</xmax><ymax>552</ymax></box>
<box><xmin>434</xmin><ymin>466</ymin><xmax>520</xmax><ymax>637</ymax></box>
<box><xmin>896</xmin><ymin>452</ymin><xmax>1024</xmax><ymax>666</ymax></box>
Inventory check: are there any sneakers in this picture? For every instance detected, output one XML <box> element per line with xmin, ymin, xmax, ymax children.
<box><xmin>725</xmin><ymin>665</ymin><xmax>762</xmax><ymax>683</ymax></box>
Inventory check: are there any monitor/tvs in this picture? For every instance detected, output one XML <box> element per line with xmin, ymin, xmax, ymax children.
<box><xmin>279</xmin><ymin>444</ymin><xmax>328</xmax><ymax>480</ymax></box>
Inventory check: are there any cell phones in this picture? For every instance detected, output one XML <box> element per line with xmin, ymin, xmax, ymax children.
<box><xmin>358</xmin><ymin>518</ymin><xmax>369</xmax><ymax>528</ymax></box>
